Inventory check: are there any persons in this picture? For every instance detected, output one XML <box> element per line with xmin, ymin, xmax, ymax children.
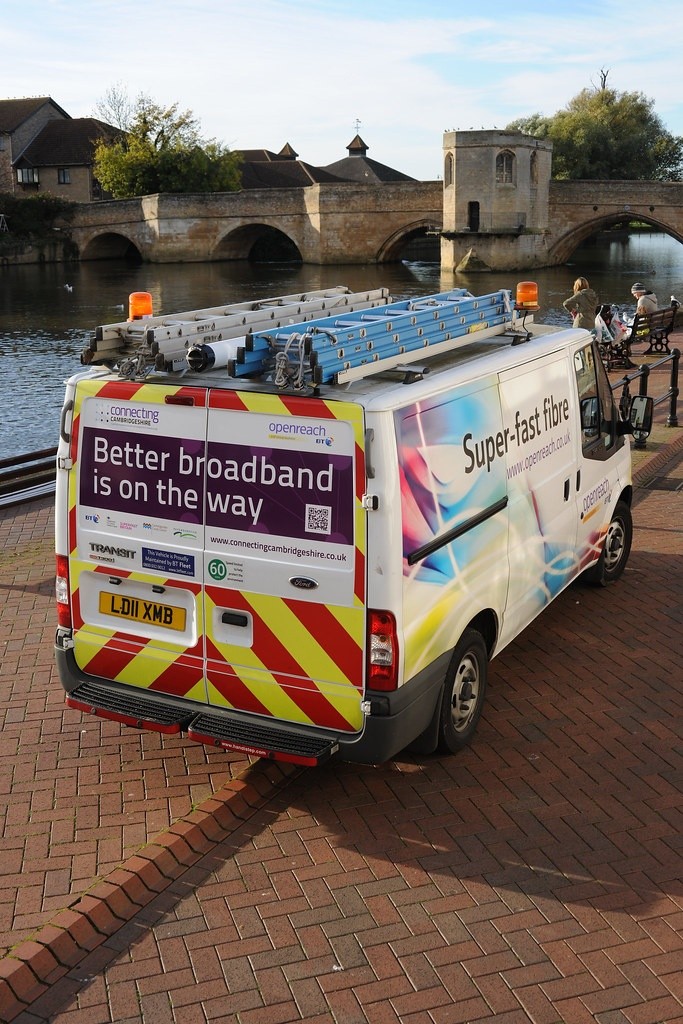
<box><xmin>631</xmin><ymin>283</ymin><xmax>657</xmax><ymax>315</ymax></box>
<box><xmin>563</xmin><ymin>277</ymin><xmax>598</xmax><ymax>334</ymax></box>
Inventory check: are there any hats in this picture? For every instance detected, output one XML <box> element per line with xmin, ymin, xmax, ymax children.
<box><xmin>631</xmin><ymin>283</ymin><xmax>646</xmax><ymax>293</ymax></box>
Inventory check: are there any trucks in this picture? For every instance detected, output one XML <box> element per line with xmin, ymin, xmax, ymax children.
<box><xmin>53</xmin><ymin>281</ymin><xmax>653</xmax><ymax>769</ymax></box>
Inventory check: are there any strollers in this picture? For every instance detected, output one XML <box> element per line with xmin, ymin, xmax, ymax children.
<box><xmin>594</xmin><ymin>304</ymin><xmax>632</xmax><ymax>372</ymax></box>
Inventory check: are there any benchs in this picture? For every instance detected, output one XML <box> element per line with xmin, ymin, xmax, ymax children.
<box><xmin>601</xmin><ymin>301</ymin><xmax>681</xmax><ymax>370</ymax></box>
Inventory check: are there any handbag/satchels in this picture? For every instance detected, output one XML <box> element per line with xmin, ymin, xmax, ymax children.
<box><xmin>594</xmin><ymin>305</ymin><xmax>615</xmax><ymax>343</ymax></box>
<box><xmin>610</xmin><ymin>312</ymin><xmax>627</xmax><ymax>346</ymax></box>
